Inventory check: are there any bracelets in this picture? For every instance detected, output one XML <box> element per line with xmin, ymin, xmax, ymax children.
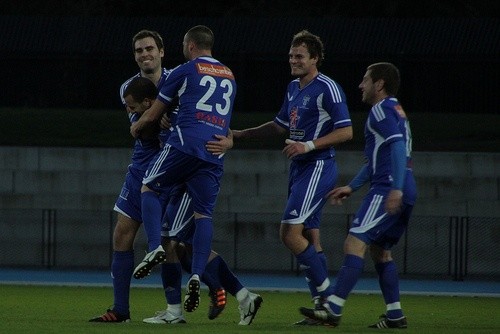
<box><xmin>307</xmin><ymin>140</ymin><xmax>316</xmax><ymax>151</ymax></box>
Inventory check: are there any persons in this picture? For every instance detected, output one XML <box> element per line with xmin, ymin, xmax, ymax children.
<box><xmin>235</xmin><ymin>31</ymin><xmax>352</xmax><ymax>325</ymax></box>
<box><xmin>123</xmin><ymin>76</ymin><xmax>263</xmax><ymax>326</ymax></box>
<box><xmin>90</xmin><ymin>31</ymin><xmax>227</xmax><ymax>323</ymax></box>
<box><xmin>130</xmin><ymin>25</ymin><xmax>235</xmax><ymax>312</ymax></box>
<box><xmin>300</xmin><ymin>63</ymin><xmax>417</xmax><ymax>328</ymax></box>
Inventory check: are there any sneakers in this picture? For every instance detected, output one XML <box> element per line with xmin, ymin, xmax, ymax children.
<box><xmin>133</xmin><ymin>244</ymin><xmax>167</xmax><ymax>280</ymax></box>
<box><xmin>88</xmin><ymin>303</ymin><xmax>131</xmax><ymax>323</ymax></box>
<box><xmin>293</xmin><ymin>308</ymin><xmax>329</xmax><ymax>326</ymax></box>
<box><xmin>143</xmin><ymin>310</ymin><xmax>187</xmax><ymax>324</ymax></box>
<box><xmin>367</xmin><ymin>314</ymin><xmax>407</xmax><ymax>329</ymax></box>
<box><xmin>184</xmin><ymin>274</ymin><xmax>201</xmax><ymax>313</ymax></box>
<box><xmin>299</xmin><ymin>307</ymin><xmax>342</xmax><ymax>327</ymax></box>
<box><xmin>208</xmin><ymin>286</ymin><xmax>228</xmax><ymax>320</ymax></box>
<box><xmin>237</xmin><ymin>291</ymin><xmax>263</xmax><ymax>326</ymax></box>
